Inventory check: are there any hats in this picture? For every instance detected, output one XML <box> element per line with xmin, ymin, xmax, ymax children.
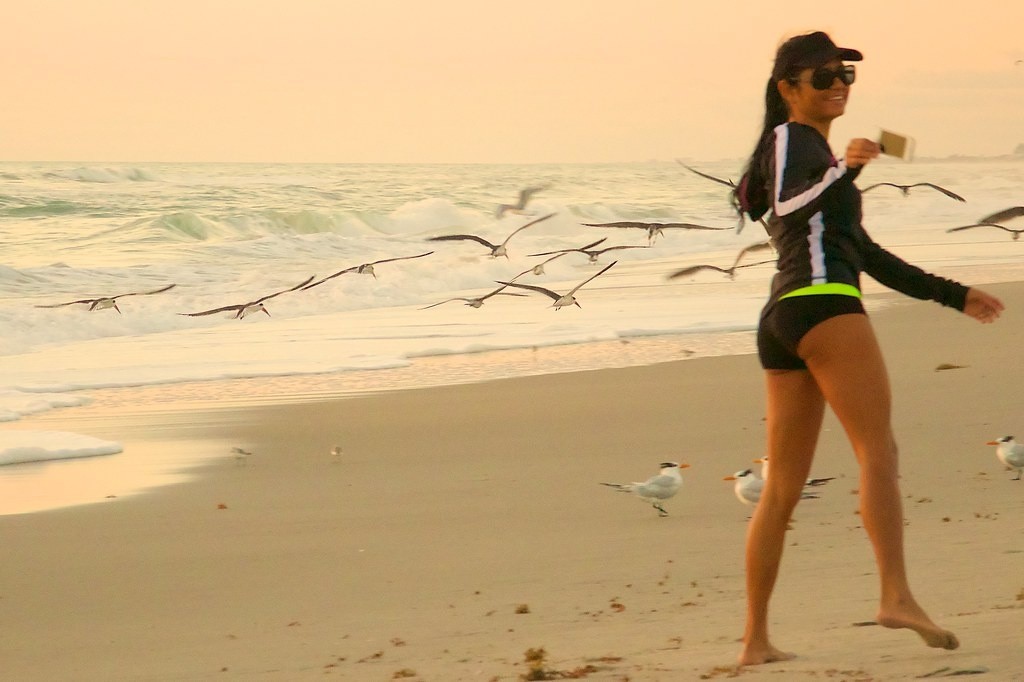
<box><xmin>772</xmin><ymin>31</ymin><xmax>863</xmax><ymax>84</ymax></box>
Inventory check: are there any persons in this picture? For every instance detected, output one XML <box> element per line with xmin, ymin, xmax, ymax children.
<box><xmin>729</xmin><ymin>31</ymin><xmax>1006</xmax><ymax>667</ymax></box>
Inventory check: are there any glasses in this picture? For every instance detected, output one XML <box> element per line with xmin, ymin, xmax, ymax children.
<box><xmin>788</xmin><ymin>65</ymin><xmax>855</xmax><ymax>90</ymax></box>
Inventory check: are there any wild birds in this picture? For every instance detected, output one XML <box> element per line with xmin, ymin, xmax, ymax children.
<box><xmin>31</xmin><ymin>161</ymin><xmax>1024</xmax><ymax>321</ymax></box>
<box><xmin>722</xmin><ymin>454</ymin><xmax>837</xmax><ymax>529</ymax></box>
<box><xmin>985</xmin><ymin>435</ymin><xmax>1024</xmax><ymax>481</ymax></box>
<box><xmin>600</xmin><ymin>462</ymin><xmax>691</xmax><ymax>517</ymax></box>
